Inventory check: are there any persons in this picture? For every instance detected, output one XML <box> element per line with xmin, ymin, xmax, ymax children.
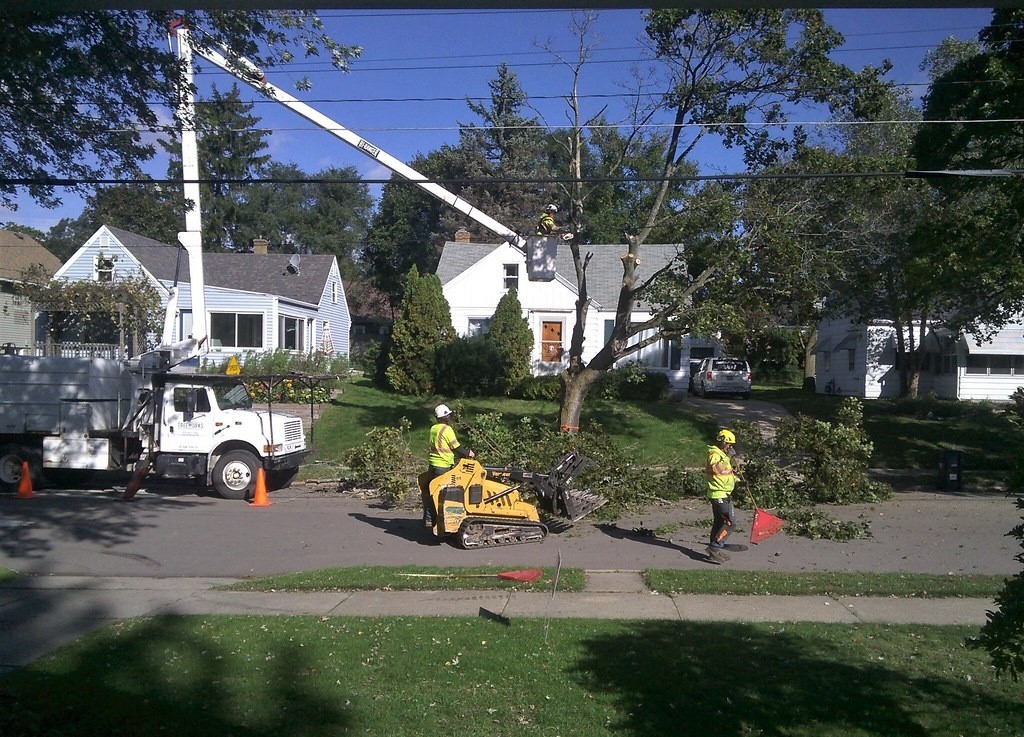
<box><xmin>702</xmin><ymin>430</ymin><xmax>736</xmax><ymax>565</ymax></box>
<box><xmin>423</xmin><ymin>405</ymin><xmax>475</xmax><ymax>527</ymax></box>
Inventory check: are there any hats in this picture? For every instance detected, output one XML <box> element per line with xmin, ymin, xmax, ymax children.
<box><xmin>434</xmin><ymin>403</ymin><xmax>453</xmax><ymax>418</ymax></box>
<box><xmin>716</xmin><ymin>428</ymin><xmax>736</xmax><ymax>444</ymax></box>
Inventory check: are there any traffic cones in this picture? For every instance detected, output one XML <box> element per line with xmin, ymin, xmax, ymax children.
<box><xmin>11</xmin><ymin>461</ymin><xmax>40</xmax><ymax>499</ymax></box>
<box><xmin>247</xmin><ymin>468</ymin><xmax>274</xmax><ymax>507</ymax></box>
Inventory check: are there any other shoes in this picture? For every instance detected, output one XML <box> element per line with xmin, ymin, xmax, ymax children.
<box><xmin>424</xmin><ymin>519</ymin><xmax>433</xmax><ymax>528</ymax></box>
<box><xmin>704</xmin><ymin>547</ymin><xmax>725</xmax><ymax>563</ymax></box>
<box><xmin>716</xmin><ymin>551</ymin><xmax>731</xmax><ymax>562</ymax></box>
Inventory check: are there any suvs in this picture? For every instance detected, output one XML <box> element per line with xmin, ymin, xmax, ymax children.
<box><xmin>692</xmin><ymin>357</ymin><xmax>752</xmax><ymax>400</ymax></box>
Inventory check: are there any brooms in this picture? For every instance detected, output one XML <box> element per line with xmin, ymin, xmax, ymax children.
<box><xmin>393</xmin><ymin>569</ymin><xmax>543</xmax><ymax>582</ymax></box>
<box><xmin>733</xmin><ymin>455</ymin><xmax>785</xmax><ymax>544</ymax></box>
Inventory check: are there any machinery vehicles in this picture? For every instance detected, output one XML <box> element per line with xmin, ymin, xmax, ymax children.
<box><xmin>417</xmin><ymin>450</ymin><xmax>609</xmax><ymax>550</ymax></box>
<box><xmin>0</xmin><ymin>17</ymin><xmax>566</xmax><ymax>500</ymax></box>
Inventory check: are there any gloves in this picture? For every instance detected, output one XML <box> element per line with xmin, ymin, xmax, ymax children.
<box><xmin>726</xmin><ymin>448</ymin><xmax>736</xmax><ymax>458</ymax></box>
<box><xmin>738</xmin><ymin>481</ymin><xmax>748</xmax><ymax>488</ymax></box>
<box><xmin>468</xmin><ymin>450</ymin><xmax>475</xmax><ymax>458</ymax></box>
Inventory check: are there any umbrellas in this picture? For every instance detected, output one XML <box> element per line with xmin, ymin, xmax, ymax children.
<box><xmin>318</xmin><ymin>323</ymin><xmax>335</xmax><ymax>359</ymax></box>
